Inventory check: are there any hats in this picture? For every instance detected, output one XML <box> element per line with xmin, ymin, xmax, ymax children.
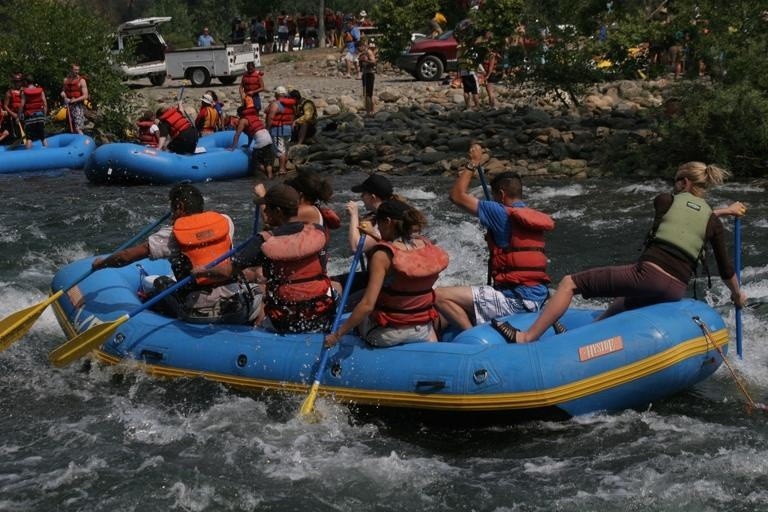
<box><xmin>201</xmin><ymin>94</ymin><xmax>214</xmax><ymax>105</ymax></box>
<box><xmin>255</xmin><ymin>184</ymin><xmax>300</xmax><ymax>204</ymax></box>
<box><xmin>351</xmin><ymin>174</ymin><xmax>393</xmax><ymax>197</ymax></box>
<box><xmin>271</xmin><ymin>87</ymin><xmax>287</xmax><ymax>94</ymax></box>
<box><xmin>360</xmin><ymin>10</ymin><xmax>367</xmax><ymax>16</ymax></box>
<box><xmin>376</xmin><ymin>201</ymin><xmax>409</xmax><ymax>219</ymax></box>
<box><xmin>14</xmin><ymin>73</ymin><xmax>23</xmax><ymax>80</ymax></box>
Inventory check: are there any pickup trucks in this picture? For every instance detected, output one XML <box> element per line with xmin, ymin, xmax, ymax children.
<box><xmin>393</xmin><ymin>25</ymin><xmax>557</xmax><ymax>82</ymax></box>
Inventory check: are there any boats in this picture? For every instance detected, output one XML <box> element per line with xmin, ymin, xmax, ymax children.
<box><xmin>1</xmin><ymin>134</ymin><xmax>96</xmax><ymax>173</ymax></box>
<box><xmin>0</xmin><ymin>131</ymin><xmax>95</xmax><ymax>180</ymax></box>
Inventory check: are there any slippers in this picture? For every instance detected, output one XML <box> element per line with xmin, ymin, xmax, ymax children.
<box><xmin>492</xmin><ymin>317</ymin><xmax>522</xmax><ymax>345</ymax></box>
<box><xmin>554</xmin><ymin>319</ymin><xmax>567</xmax><ymax>335</ymax></box>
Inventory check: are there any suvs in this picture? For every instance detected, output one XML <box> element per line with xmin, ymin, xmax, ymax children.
<box><xmin>96</xmin><ymin>15</ymin><xmax>170</xmax><ymax>87</ymax></box>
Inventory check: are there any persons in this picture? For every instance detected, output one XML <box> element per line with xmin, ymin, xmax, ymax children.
<box><xmin>206</xmin><ymin>91</ymin><xmax>224</xmax><ymax>132</ymax></box>
<box><xmin>195</xmin><ymin>93</ymin><xmax>220</xmax><ymax>138</ymax></box>
<box><xmin>483</xmin><ymin>44</ymin><xmax>497</xmax><ymax>111</ymax></box>
<box><xmin>20</xmin><ymin>75</ymin><xmax>48</xmax><ymax>150</ymax></box>
<box><xmin>236</xmin><ymin>173</ymin><xmax>335</xmax><ymax>302</ymax></box>
<box><xmin>505</xmin><ymin>28</ymin><xmax>527</xmax><ymax>84</ymax></box>
<box><xmin>342</xmin><ymin>16</ymin><xmax>363</xmax><ymax>82</ymax></box>
<box><xmin>241</xmin><ymin>63</ymin><xmax>264</xmax><ymax>121</ymax></box>
<box><xmin>458</xmin><ymin>38</ymin><xmax>486</xmax><ymax>112</ymax></box>
<box><xmin>4</xmin><ymin>76</ymin><xmax>24</xmax><ymax>146</ymax></box>
<box><xmin>330</xmin><ymin>175</ymin><xmax>395</xmax><ymax>314</ymax></box>
<box><xmin>325</xmin><ymin>199</ymin><xmax>449</xmax><ymax>347</ymax></box>
<box><xmin>698</xmin><ymin>19</ymin><xmax>712</xmax><ymax>77</ymax></box>
<box><xmin>430</xmin><ymin>12</ymin><xmax>447</xmax><ymax>40</ymax></box>
<box><xmin>136</xmin><ymin>111</ymin><xmax>160</xmax><ymax>147</ymax></box>
<box><xmin>225</xmin><ymin>106</ymin><xmax>275</xmax><ymax>180</ymax></box>
<box><xmin>155</xmin><ymin>107</ymin><xmax>198</xmax><ymax>155</ymax></box>
<box><xmin>189</xmin><ymin>184</ymin><xmax>341</xmax><ymax>334</ymax></box>
<box><xmin>491</xmin><ymin>163</ymin><xmax>748</xmax><ymax>345</ymax></box>
<box><xmin>667</xmin><ymin>16</ymin><xmax>685</xmax><ymax>80</ymax></box>
<box><xmin>0</xmin><ymin>101</ymin><xmax>4</xmax><ymax>125</ymax></box>
<box><xmin>0</xmin><ymin>111</ymin><xmax>14</xmax><ymax>146</ymax></box>
<box><xmin>60</xmin><ymin>63</ymin><xmax>88</xmax><ymax>134</ymax></box>
<box><xmin>434</xmin><ymin>145</ymin><xmax>553</xmax><ymax>335</ymax></box>
<box><xmin>93</xmin><ymin>183</ymin><xmax>263</xmax><ymax>327</ymax></box>
<box><xmin>230</xmin><ymin>9</ymin><xmax>346</xmax><ymax>55</ymax></box>
<box><xmin>197</xmin><ymin>26</ymin><xmax>215</xmax><ymax>48</ymax></box>
<box><xmin>265</xmin><ymin>87</ymin><xmax>295</xmax><ymax>175</ymax></box>
<box><xmin>355</xmin><ymin>40</ymin><xmax>376</xmax><ymax>119</ymax></box>
<box><xmin>535</xmin><ymin>19</ymin><xmax>547</xmax><ymax>64</ymax></box>
<box><xmin>633</xmin><ymin>43</ymin><xmax>653</xmax><ymax>82</ymax></box>
<box><xmin>289</xmin><ymin>89</ymin><xmax>318</xmax><ymax>145</ymax></box>
<box><xmin>359</xmin><ymin>11</ymin><xmax>368</xmax><ymax>37</ymax></box>
<box><xmin>641</xmin><ymin>8</ymin><xmax>668</xmax><ymax>82</ymax></box>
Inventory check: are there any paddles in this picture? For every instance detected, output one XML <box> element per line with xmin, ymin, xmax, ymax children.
<box><xmin>297</xmin><ymin>224</ymin><xmax>367</xmax><ymax>422</ymax></box>
<box><xmin>50</xmin><ymin>226</ymin><xmax>268</xmax><ymax>364</ymax></box>
<box><xmin>8</xmin><ymin>133</ymin><xmax>30</xmax><ymax>149</ymax></box>
<box><xmin>17</xmin><ymin>121</ymin><xmax>27</xmax><ymax>145</ymax></box>
<box><xmin>0</xmin><ymin>213</ymin><xmax>170</xmax><ymax>354</ymax></box>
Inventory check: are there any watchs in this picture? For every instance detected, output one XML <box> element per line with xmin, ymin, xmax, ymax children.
<box><xmin>467</xmin><ymin>162</ymin><xmax>479</xmax><ymax>173</ymax></box>
<box><xmin>333</xmin><ymin>332</ymin><xmax>342</xmax><ymax>340</ymax></box>
<box><xmin>104</xmin><ymin>259</ymin><xmax>107</xmax><ymax>268</ymax></box>
<box><xmin>207</xmin><ymin>270</ymin><xmax>212</xmax><ymax>279</ymax></box>
